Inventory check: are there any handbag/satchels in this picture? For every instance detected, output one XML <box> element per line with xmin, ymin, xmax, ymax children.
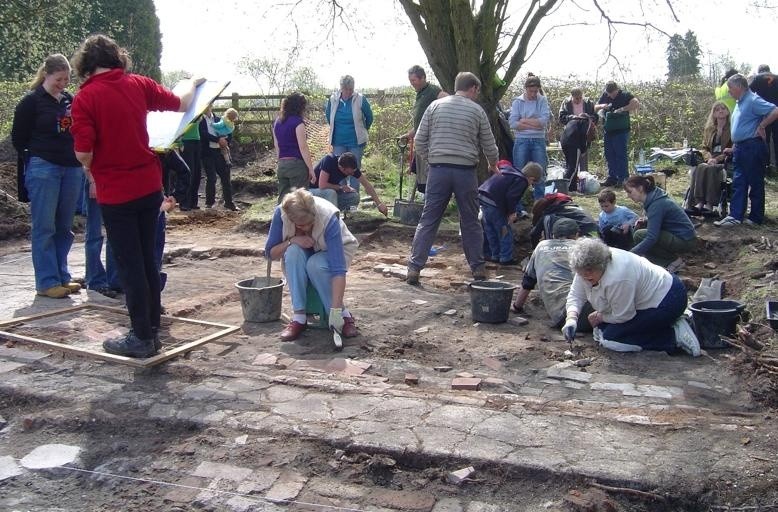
<box><xmin>604</xmin><ymin>110</ymin><xmax>630</xmax><ymax>134</ymax></box>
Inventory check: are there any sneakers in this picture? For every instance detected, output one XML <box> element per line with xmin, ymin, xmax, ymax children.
<box><xmin>179</xmin><ymin>205</ymin><xmax>190</xmax><ymax>212</ymax></box>
<box><xmin>154</xmin><ymin>331</ymin><xmax>163</xmax><ymax>352</ymax></box>
<box><xmin>743</xmin><ymin>218</ymin><xmax>761</xmax><ymax>225</ymax></box>
<box><xmin>87</xmin><ymin>287</ymin><xmax>116</xmax><ymax>299</ymax></box>
<box><xmin>342</xmin><ymin>312</ymin><xmax>357</xmax><ymax>338</ymax></box>
<box><xmin>406</xmin><ymin>267</ymin><xmax>420</xmax><ymax>286</ymax></box>
<box><xmin>204</xmin><ymin>203</ymin><xmax>215</xmax><ymax>210</ymax></box>
<box><xmin>702</xmin><ymin>208</ymin><xmax>713</xmax><ymax>216</ymax></box>
<box><xmin>712</xmin><ymin>215</ymin><xmax>742</xmax><ymax>228</ymax></box>
<box><xmin>280</xmin><ymin>320</ymin><xmax>308</xmax><ymax>340</ymax></box>
<box><xmin>104</xmin><ymin>330</ymin><xmax>156</xmax><ymax>357</ymax></box>
<box><xmin>224</xmin><ymin>202</ymin><xmax>241</xmax><ymax>211</ymax></box>
<box><xmin>187</xmin><ymin>204</ymin><xmax>201</xmax><ymax>209</ymax></box>
<box><xmin>671</xmin><ymin>318</ymin><xmax>701</xmax><ymax>358</ymax></box>
<box><xmin>36</xmin><ymin>285</ymin><xmax>70</xmax><ymax>298</ymax></box>
<box><xmin>685</xmin><ymin>207</ymin><xmax>701</xmax><ymax>216</ymax></box>
<box><xmin>599</xmin><ymin>177</ymin><xmax>617</xmax><ymax>187</ymax></box>
<box><xmin>499</xmin><ymin>257</ymin><xmax>519</xmax><ymax>266</ymax></box>
<box><xmin>62</xmin><ymin>282</ymin><xmax>81</xmax><ymax>292</ymax></box>
<box><xmin>471</xmin><ymin>270</ymin><xmax>488</xmax><ymax>281</ymax></box>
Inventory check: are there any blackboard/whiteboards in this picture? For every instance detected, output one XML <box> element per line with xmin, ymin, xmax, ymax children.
<box><xmin>147</xmin><ymin>78</ymin><xmax>231</xmax><ymax>154</ymax></box>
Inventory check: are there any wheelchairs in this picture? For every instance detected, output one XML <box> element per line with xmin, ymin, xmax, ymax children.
<box><xmin>678</xmin><ymin>148</ymin><xmax>749</xmax><ymax>219</ymax></box>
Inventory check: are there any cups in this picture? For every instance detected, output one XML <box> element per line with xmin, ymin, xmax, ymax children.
<box><xmin>675</xmin><ymin>143</ymin><xmax>680</xmax><ymax>148</ymax></box>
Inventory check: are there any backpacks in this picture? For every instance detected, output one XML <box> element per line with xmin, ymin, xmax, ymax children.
<box><xmin>531</xmin><ymin>190</ymin><xmax>574</xmax><ymax>226</ymax></box>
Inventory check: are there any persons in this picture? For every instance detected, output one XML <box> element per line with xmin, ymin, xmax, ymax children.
<box><xmin>171</xmin><ymin>103</ymin><xmax>240</xmax><ymax>210</ymax></box>
<box><xmin>70</xmin><ymin>34</ymin><xmax>206</xmax><ymax>358</ymax></box>
<box><xmin>510</xmin><ymin>174</ymin><xmax>701</xmax><ymax>355</ymax></box>
<box><xmin>559</xmin><ymin>89</ymin><xmax>599</xmax><ymax>192</ymax></box>
<box><xmin>263</xmin><ymin>187</ymin><xmax>360</xmax><ymax>342</ymax></box>
<box><xmin>273</xmin><ymin>75</ymin><xmax>390</xmax><ymax>217</ymax></box>
<box><xmin>12</xmin><ymin>53</ymin><xmax>85</xmax><ymax>298</ymax></box>
<box><xmin>81</xmin><ymin>148</ymin><xmax>192</xmax><ymax>297</ymax></box>
<box><xmin>714</xmin><ymin>67</ymin><xmax>776</xmax><ymax>178</ymax></box>
<box><xmin>595</xmin><ymin>81</ymin><xmax>639</xmax><ymax>188</ymax></box>
<box><xmin>405</xmin><ymin>71</ymin><xmax>499</xmax><ymax>283</ymax></box>
<box><xmin>685</xmin><ymin>101</ymin><xmax>732</xmax><ymax>217</ymax></box>
<box><xmin>510</xmin><ymin>73</ymin><xmax>554</xmax><ymax>202</ymax></box>
<box><xmin>477</xmin><ymin>161</ymin><xmax>543</xmax><ymax>264</ymax></box>
<box><xmin>400</xmin><ymin>68</ymin><xmax>450</xmax><ymax>194</ymax></box>
<box><xmin>714</xmin><ymin>74</ymin><xmax>776</xmax><ymax>226</ymax></box>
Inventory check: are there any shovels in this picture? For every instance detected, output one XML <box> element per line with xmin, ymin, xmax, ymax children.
<box><xmin>251</xmin><ymin>259</ymin><xmax>281</xmax><ymax>288</ymax></box>
<box><xmin>393</xmin><ymin>138</ymin><xmax>406</xmax><ymax>216</ymax></box>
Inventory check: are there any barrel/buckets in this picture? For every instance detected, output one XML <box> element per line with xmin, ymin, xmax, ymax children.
<box><xmin>235</xmin><ymin>279</ymin><xmax>285</xmax><ymax>323</ymax></box>
<box><xmin>398</xmin><ymin>199</ymin><xmax>424</xmax><ymax>225</ymax></box>
<box><xmin>468</xmin><ymin>280</ymin><xmax>514</xmax><ymax>324</ymax></box>
<box><xmin>689</xmin><ymin>300</ymin><xmax>745</xmax><ymax>347</ymax></box>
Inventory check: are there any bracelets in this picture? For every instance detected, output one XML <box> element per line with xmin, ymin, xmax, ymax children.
<box><xmin>287</xmin><ymin>237</ymin><xmax>293</xmax><ymax>245</ymax></box>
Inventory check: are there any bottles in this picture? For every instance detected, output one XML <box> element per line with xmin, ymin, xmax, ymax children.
<box><xmin>683</xmin><ymin>138</ymin><xmax>688</xmax><ymax>149</ymax></box>
<box><xmin>639</xmin><ymin>149</ymin><xmax>646</xmax><ymax>167</ymax></box>
<box><xmin>576</xmin><ymin>177</ymin><xmax>580</xmax><ymax>191</ymax></box>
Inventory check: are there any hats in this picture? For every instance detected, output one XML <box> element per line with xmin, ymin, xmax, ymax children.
<box><xmin>551</xmin><ymin>218</ymin><xmax>580</xmax><ymax>234</ymax></box>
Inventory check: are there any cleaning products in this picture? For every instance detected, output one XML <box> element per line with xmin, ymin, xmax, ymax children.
<box><xmin>683</xmin><ymin>137</ymin><xmax>688</xmax><ymax>149</ymax></box>
